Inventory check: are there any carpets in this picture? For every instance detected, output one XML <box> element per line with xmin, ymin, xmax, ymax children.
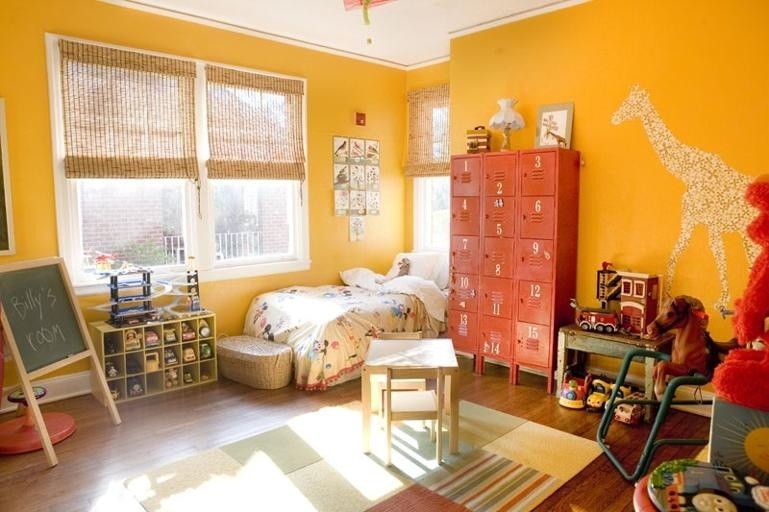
<box><xmin>123</xmin><ymin>399</ymin><xmax>612</xmax><ymax>512</ymax></box>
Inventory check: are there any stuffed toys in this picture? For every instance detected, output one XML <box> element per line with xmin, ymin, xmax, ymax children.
<box><xmin>373</xmin><ymin>256</ymin><xmax>412</xmax><ymax>284</ymax></box>
<box><xmin>719</xmin><ymin>169</ymin><xmax>768</xmax><ymax>408</ymax></box>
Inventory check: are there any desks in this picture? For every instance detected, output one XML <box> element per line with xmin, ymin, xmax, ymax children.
<box><xmin>356</xmin><ymin>338</ymin><xmax>463</xmax><ymax>456</ymax></box>
<box><xmin>556</xmin><ymin>318</ymin><xmax>674</xmax><ymax>422</ymax></box>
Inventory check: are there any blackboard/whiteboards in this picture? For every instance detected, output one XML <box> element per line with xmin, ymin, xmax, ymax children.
<box><xmin>0</xmin><ymin>256</ymin><xmax>94</xmax><ymax>381</ymax></box>
<box><xmin>0</xmin><ymin>97</ymin><xmax>16</xmax><ymax>256</ymax></box>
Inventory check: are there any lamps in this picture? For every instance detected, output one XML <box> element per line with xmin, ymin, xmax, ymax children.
<box><xmin>488</xmin><ymin>93</ymin><xmax>526</xmax><ymax>151</ymax></box>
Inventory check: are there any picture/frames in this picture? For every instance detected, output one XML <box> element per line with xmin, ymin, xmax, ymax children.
<box><xmin>533</xmin><ymin>102</ymin><xmax>575</xmax><ymax>149</ymax></box>
<box><xmin>0</xmin><ymin>94</ymin><xmax>19</xmax><ymax>258</ymax></box>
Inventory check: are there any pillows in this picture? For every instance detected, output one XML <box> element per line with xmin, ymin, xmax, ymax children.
<box><xmin>391</xmin><ymin>252</ymin><xmax>449</xmax><ymax>290</ymax></box>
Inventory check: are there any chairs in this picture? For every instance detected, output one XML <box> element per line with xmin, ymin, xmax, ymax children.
<box><xmin>377</xmin><ymin>331</ymin><xmax>429</xmax><ymax>429</ymax></box>
<box><xmin>380</xmin><ymin>364</ymin><xmax>445</xmax><ymax>466</ymax></box>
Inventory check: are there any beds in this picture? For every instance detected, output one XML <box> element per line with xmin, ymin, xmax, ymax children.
<box><xmin>243</xmin><ymin>249</ymin><xmax>450</xmax><ymax>391</ymax></box>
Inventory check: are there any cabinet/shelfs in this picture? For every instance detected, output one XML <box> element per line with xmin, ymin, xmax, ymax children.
<box><xmin>102</xmin><ymin>264</ymin><xmax>158</xmax><ymax>329</ymax></box>
<box><xmin>87</xmin><ymin>306</ymin><xmax>218</xmax><ymax>406</ymax></box>
<box><xmin>449</xmin><ymin>146</ymin><xmax>580</xmax><ymax>394</ymax></box>
